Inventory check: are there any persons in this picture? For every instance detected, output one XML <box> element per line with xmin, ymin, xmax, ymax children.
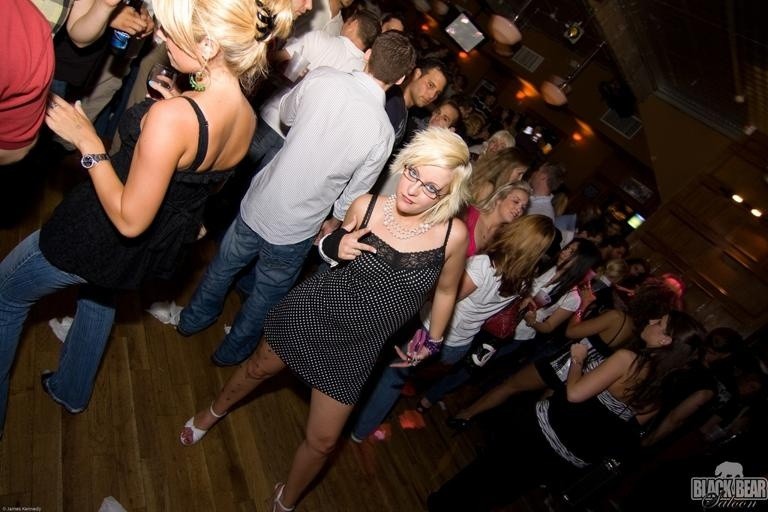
<box><xmin>0</xmin><ymin>1</ymin><xmax>768</xmax><ymax>512</ymax></box>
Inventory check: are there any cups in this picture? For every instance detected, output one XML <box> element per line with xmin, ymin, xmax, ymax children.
<box><xmin>527</xmin><ymin>291</ymin><xmax>552</xmax><ymax>312</ymax></box>
<box><xmin>146</xmin><ymin>64</ymin><xmax>178</xmax><ymax>100</ymax></box>
<box><xmin>283</xmin><ymin>52</ymin><xmax>311</xmax><ymax>82</ymax></box>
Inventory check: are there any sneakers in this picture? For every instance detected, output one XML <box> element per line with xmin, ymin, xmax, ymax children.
<box><xmin>42</xmin><ymin>370</ymin><xmax>85</xmax><ymax>414</ymax></box>
<box><xmin>49</xmin><ymin>316</ymin><xmax>75</xmax><ymax>342</ymax></box>
<box><xmin>149</xmin><ymin>301</ymin><xmax>183</xmax><ymax>325</ymax></box>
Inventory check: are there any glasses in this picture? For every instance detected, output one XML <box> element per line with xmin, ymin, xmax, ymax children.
<box><xmin>403</xmin><ymin>167</ymin><xmax>445</xmax><ymax>200</ymax></box>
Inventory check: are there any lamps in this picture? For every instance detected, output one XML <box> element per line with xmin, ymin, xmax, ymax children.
<box><xmin>488</xmin><ymin>1</ymin><xmax>533</xmax><ymax>48</ymax></box>
<box><xmin>539</xmin><ymin>36</ymin><xmax>608</xmax><ymax>110</ymax></box>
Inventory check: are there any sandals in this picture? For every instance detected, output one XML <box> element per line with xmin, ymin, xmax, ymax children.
<box><xmin>271</xmin><ymin>482</ymin><xmax>294</xmax><ymax>511</ymax></box>
<box><xmin>181</xmin><ymin>402</ymin><xmax>225</xmax><ymax>446</ymax></box>
<box><xmin>416</xmin><ymin>398</ymin><xmax>431</xmax><ymax>414</ymax></box>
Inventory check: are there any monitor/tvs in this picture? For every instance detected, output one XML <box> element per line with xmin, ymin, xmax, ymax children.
<box><xmin>442</xmin><ymin>10</ymin><xmax>487</xmax><ymax>55</ymax></box>
